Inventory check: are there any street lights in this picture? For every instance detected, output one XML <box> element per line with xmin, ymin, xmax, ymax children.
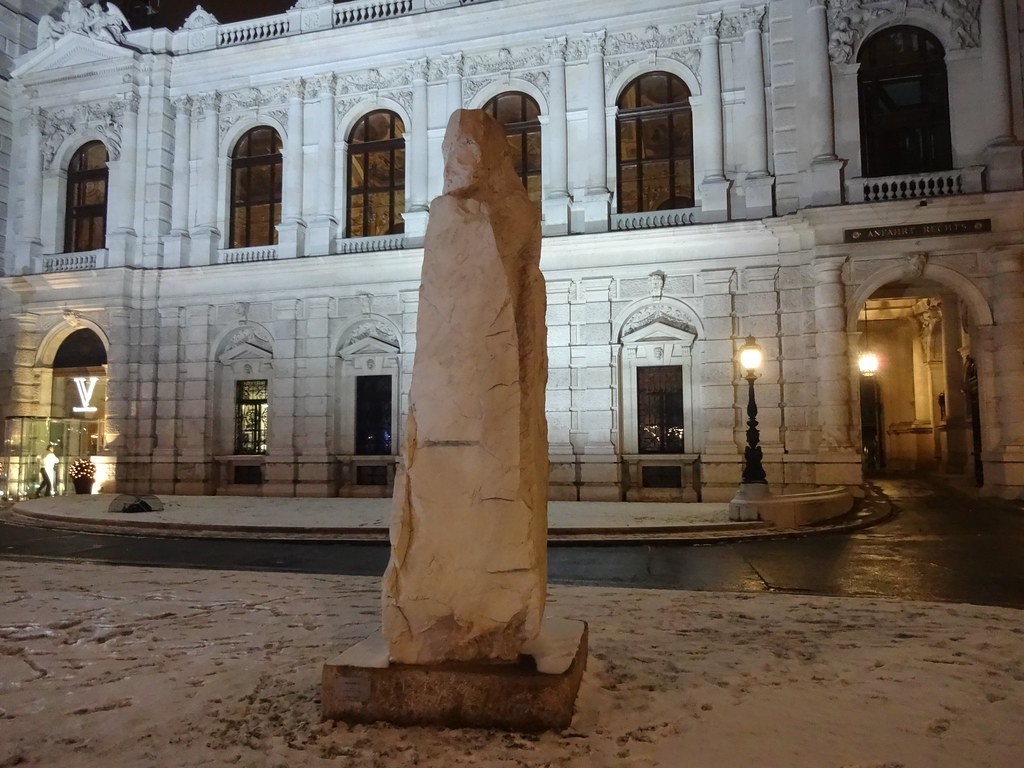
<box><xmin>736</xmin><ymin>333</ymin><xmax>769</xmax><ymax>484</ymax></box>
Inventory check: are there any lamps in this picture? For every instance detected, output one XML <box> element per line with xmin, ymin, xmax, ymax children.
<box><xmin>858</xmin><ymin>302</ymin><xmax>879</xmax><ymax>378</ymax></box>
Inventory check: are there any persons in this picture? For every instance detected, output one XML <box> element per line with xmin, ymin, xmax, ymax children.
<box><xmin>35</xmin><ymin>445</ymin><xmax>60</xmax><ymax>498</ymax></box>
